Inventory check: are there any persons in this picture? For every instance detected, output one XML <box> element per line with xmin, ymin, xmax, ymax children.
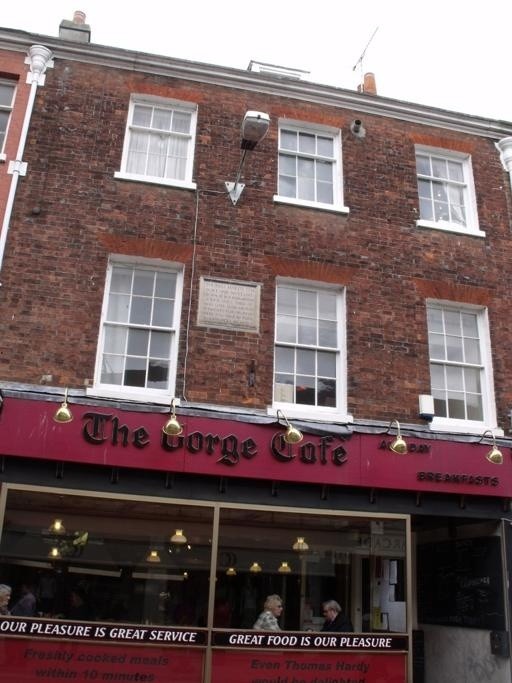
<box><xmin>319</xmin><ymin>599</ymin><xmax>354</xmax><ymax>632</ymax></box>
<box><xmin>252</xmin><ymin>594</ymin><xmax>284</xmax><ymax>631</ymax></box>
<box><xmin>0</xmin><ymin>575</ymin><xmax>242</xmax><ymax>627</ymax></box>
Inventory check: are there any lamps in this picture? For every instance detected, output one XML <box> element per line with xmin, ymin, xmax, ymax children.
<box><xmin>223</xmin><ymin>108</ymin><xmax>273</xmax><ymax>206</ymax></box>
<box><xmin>30</xmin><ymin>517</ymin><xmax>309</xmax><ymax>582</ymax></box>
<box><xmin>476</xmin><ymin>428</ymin><xmax>504</xmax><ymax>465</ymax></box>
<box><xmin>384</xmin><ymin>416</ymin><xmax>410</xmax><ymax>455</ymax></box>
<box><xmin>52</xmin><ymin>382</ymin><xmax>75</xmax><ymax>424</ymax></box>
<box><xmin>271</xmin><ymin>406</ymin><xmax>305</xmax><ymax>443</ymax></box>
<box><xmin>160</xmin><ymin>395</ymin><xmax>184</xmax><ymax>438</ymax></box>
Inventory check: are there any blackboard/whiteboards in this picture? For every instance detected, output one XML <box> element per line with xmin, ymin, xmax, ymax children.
<box><xmin>416</xmin><ymin>535</ymin><xmax>506</xmax><ymax>631</ymax></box>
